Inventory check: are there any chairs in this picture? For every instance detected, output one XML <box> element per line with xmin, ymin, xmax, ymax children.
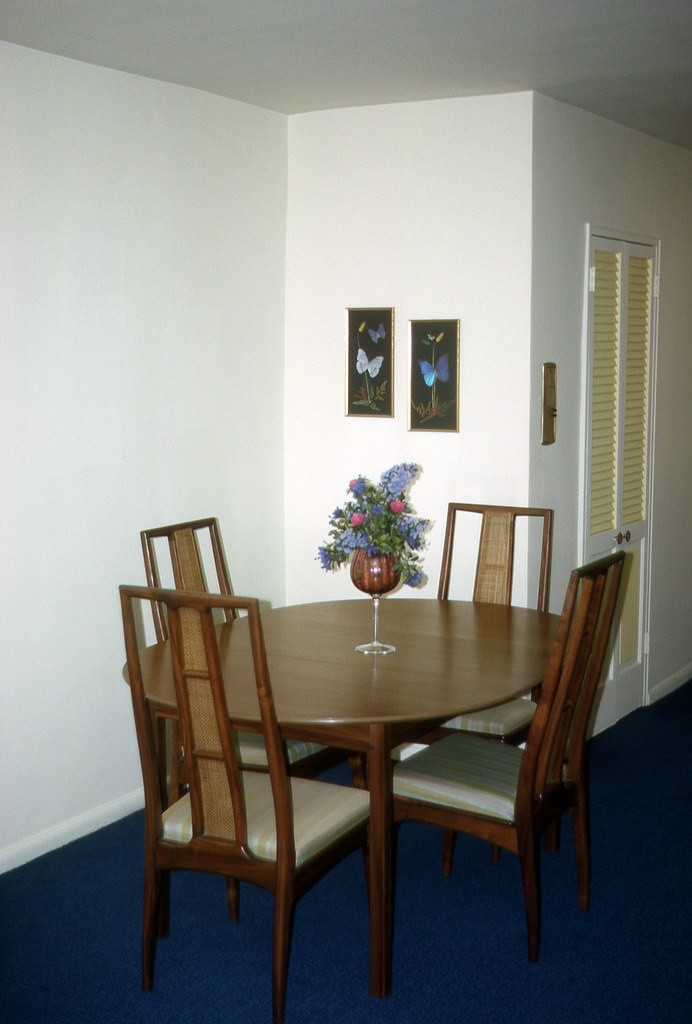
<box><xmin>392</xmin><ymin>549</ymin><xmax>627</xmax><ymax>964</ymax></box>
<box><xmin>347</xmin><ymin>501</ymin><xmax>555</xmax><ymax>881</ymax></box>
<box><xmin>119</xmin><ymin>585</ymin><xmax>368</xmax><ymax>1024</ymax></box>
<box><xmin>140</xmin><ymin>517</ymin><xmax>347</xmax><ymax>810</ymax></box>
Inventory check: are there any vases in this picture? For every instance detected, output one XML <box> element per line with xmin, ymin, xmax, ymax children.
<box><xmin>349</xmin><ymin>549</ymin><xmax>402</xmax><ymax>655</ymax></box>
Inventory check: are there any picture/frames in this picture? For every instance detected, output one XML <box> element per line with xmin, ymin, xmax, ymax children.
<box><xmin>407</xmin><ymin>317</ymin><xmax>460</xmax><ymax>435</ymax></box>
<box><xmin>344</xmin><ymin>305</ymin><xmax>398</xmax><ymax>420</ymax></box>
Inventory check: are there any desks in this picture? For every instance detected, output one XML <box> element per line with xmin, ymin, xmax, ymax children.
<box><xmin>122</xmin><ymin>599</ymin><xmax>562</xmax><ymax>1001</ymax></box>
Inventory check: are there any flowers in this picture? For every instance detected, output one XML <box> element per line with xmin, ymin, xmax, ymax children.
<box><xmin>313</xmin><ymin>463</ymin><xmax>434</xmax><ymax>588</ymax></box>
